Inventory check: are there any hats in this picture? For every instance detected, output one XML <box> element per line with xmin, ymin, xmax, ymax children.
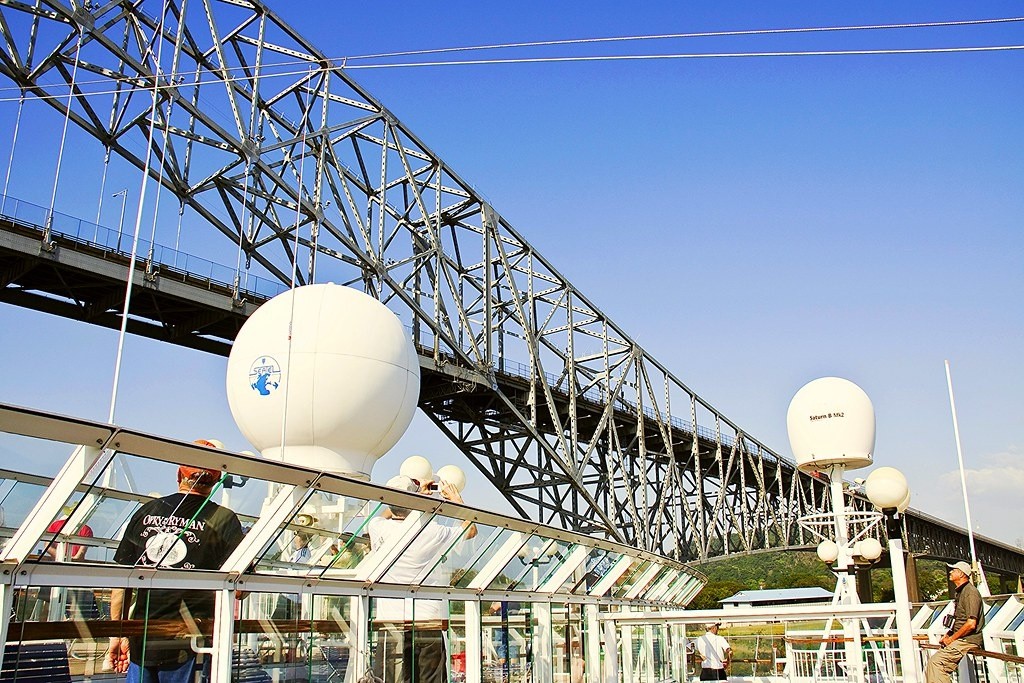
<box><xmin>180</xmin><ymin>440</ymin><xmax>220</xmax><ymax>483</ymax></box>
<box><xmin>63</xmin><ymin>502</ymin><xmax>78</xmax><ymax>515</ymax></box>
<box><xmin>947</xmin><ymin>561</ymin><xmax>972</xmax><ymax>579</ymax></box>
<box><xmin>294</xmin><ymin>531</ymin><xmax>309</xmax><ymax>539</ymax></box>
<box><xmin>386</xmin><ymin>476</ymin><xmax>418</xmax><ymax>493</ymax></box>
<box><xmin>705</xmin><ymin>623</ymin><xmax>716</xmax><ymax>628</ymax></box>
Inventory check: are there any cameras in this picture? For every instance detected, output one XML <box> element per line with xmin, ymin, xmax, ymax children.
<box><xmin>430</xmin><ymin>483</ymin><xmax>442</xmax><ymax>492</ymax></box>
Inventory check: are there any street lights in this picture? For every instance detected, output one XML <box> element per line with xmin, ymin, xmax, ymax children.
<box><xmin>789</xmin><ymin>378</ymin><xmax>876</xmax><ymax>683</ymax></box>
<box><xmin>516</xmin><ymin>538</ymin><xmax>558</xmax><ymax>633</ymax></box>
<box><xmin>865</xmin><ymin>466</ymin><xmax>917</xmax><ymax>683</ymax></box>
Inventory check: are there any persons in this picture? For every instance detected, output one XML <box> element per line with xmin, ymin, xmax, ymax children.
<box><xmin>271</xmin><ymin>531</ymin><xmax>313</xmax><ymax>637</ymax></box>
<box><xmin>488</xmin><ymin>602</ymin><xmax>521</xmax><ymax>664</ymax></box>
<box><xmin>308</xmin><ymin>532</ymin><xmax>375</xmax><ymax>619</ymax></box>
<box><xmin>368</xmin><ymin>475</ymin><xmax>478</xmax><ymax>683</ymax></box>
<box><xmin>686</xmin><ymin>623</ymin><xmax>733</xmax><ymax>681</ymax></box>
<box><xmin>101</xmin><ymin>491</ymin><xmax>164</xmax><ymax>671</ymax></box>
<box><xmin>926</xmin><ymin>561</ymin><xmax>986</xmax><ymax>683</ymax></box>
<box><xmin>36</xmin><ymin>519</ymin><xmax>100</xmax><ymax>619</ymax></box>
<box><xmin>108</xmin><ymin>439</ymin><xmax>255</xmax><ymax>683</ymax></box>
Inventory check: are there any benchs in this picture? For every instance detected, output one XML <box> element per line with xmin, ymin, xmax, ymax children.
<box><xmin>0</xmin><ymin>642</ymin><xmax>72</xmax><ymax>683</ymax></box>
<box><xmin>622</xmin><ymin>636</ymin><xmax>696</xmax><ymax>677</ymax></box>
<box><xmin>317</xmin><ymin>645</ymin><xmax>350</xmax><ymax>683</ymax></box>
<box><xmin>231</xmin><ymin>648</ymin><xmax>273</xmax><ymax>683</ymax></box>
<box><xmin>57</xmin><ymin>589</ymin><xmax>113</xmax><ymax>660</ymax></box>
